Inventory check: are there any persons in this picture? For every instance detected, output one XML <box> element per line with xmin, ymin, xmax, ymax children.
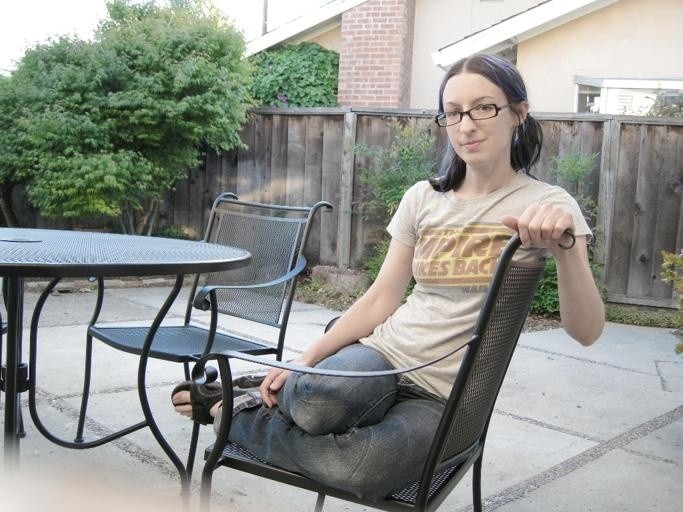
<box><xmin>170</xmin><ymin>51</ymin><xmax>610</xmax><ymax>504</ymax></box>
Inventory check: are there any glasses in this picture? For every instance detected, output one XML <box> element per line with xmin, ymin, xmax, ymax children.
<box><xmin>435</xmin><ymin>102</ymin><xmax>511</xmax><ymax>127</ymax></box>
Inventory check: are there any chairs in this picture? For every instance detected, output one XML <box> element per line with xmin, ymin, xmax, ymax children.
<box><xmin>75</xmin><ymin>192</ymin><xmax>334</xmax><ymax>483</ymax></box>
<box><xmin>191</xmin><ymin>227</ymin><xmax>576</xmax><ymax>511</ymax></box>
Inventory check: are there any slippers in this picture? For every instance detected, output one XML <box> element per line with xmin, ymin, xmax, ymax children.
<box><xmin>170</xmin><ymin>381</ymin><xmax>251</xmax><ymax>425</ymax></box>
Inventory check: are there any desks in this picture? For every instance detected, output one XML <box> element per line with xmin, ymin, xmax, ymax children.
<box><xmin>1</xmin><ymin>227</ymin><xmax>250</xmax><ymax>511</ymax></box>
